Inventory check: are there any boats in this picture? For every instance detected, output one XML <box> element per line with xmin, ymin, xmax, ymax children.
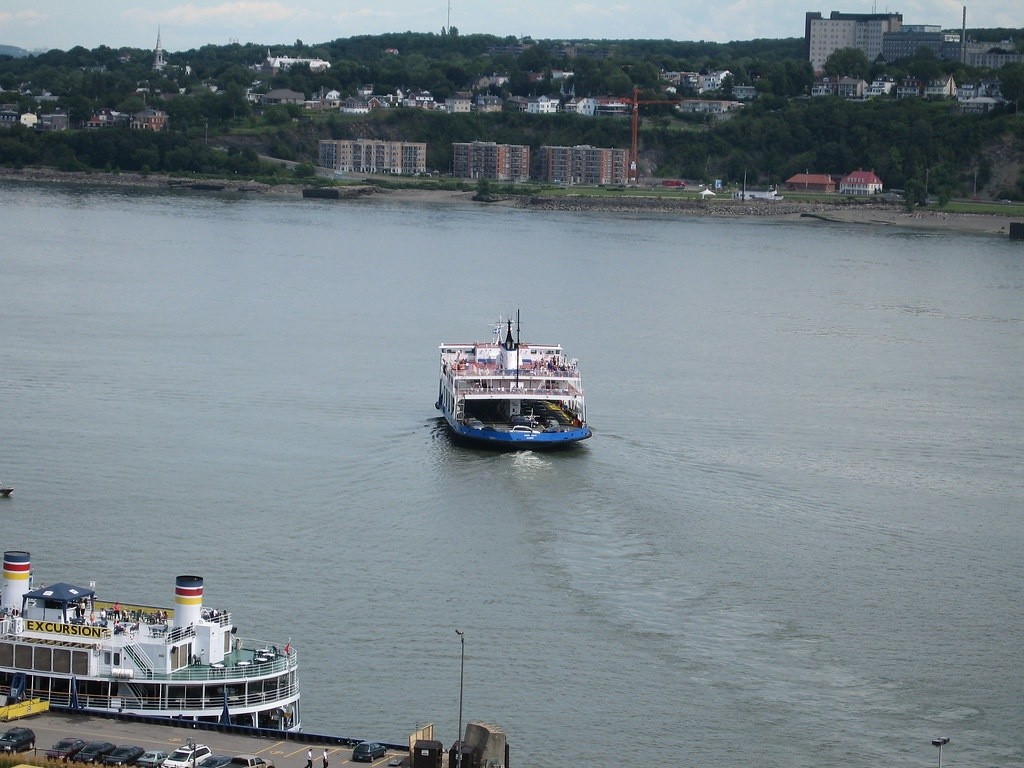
<box><xmin>433</xmin><ymin>308</ymin><xmax>594</xmax><ymax>447</ymax></box>
<box><xmin>0</xmin><ymin>483</ymin><xmax>14</xmax><ymax>496</ymax></box>
<box><xmin>0</xmin><ymin>550</ymin><xmax>304</xmax><ymax>732</ymax></box>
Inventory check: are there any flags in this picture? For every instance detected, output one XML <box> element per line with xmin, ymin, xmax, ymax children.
<box><xmin>283</xmin><ymin>642</ymin><xmax>291</xmax><ymax>654</ymax></box>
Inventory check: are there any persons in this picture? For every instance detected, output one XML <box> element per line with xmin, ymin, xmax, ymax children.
<box><xmin>451</xmin><ymin>358</ymin><xmax>573</xmax><ymax>378</ymax></box>
<box><xmin>323</xmin><ymin>748</ymin><xmax>328</xmax><ymax>768</ymax></box>
<box><xmin>305</xmin><ymin>749</ymin><xmax>313</xmax><ymax>768</ymax></box>
<box><xmin>3</xmin><ymin>583</ymin><xmax>229</xmax><ymax>644</ymax></box>
<box><xmin>253</xmin><ymin>645</ymin><xmax>278</xmax><ymax>660</ymax></box>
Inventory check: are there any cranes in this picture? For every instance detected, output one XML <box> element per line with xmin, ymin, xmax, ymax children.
<box><xmin>600</xmin><ymin>87</ymin><xmax>732</xmax><ymax>181</ymax></box>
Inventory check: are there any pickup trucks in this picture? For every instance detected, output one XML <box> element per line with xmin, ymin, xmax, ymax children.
<box><xmin>218</xmin><ymin>754</ymin><xmax>275</xmax><ymax>768</ymax></box>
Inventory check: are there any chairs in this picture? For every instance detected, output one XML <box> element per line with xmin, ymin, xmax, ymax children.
<box><xmin>210</xmin><ymin>648</ymin><xmax>278</xmax><ymax>677</ymax></box>
<box><xmin>193</xmin><ymin>654</ymin><xmax>201</xmax><ymax>666</ymax></box>
<box><xmin>152</xmin><ymin>628</ymin><xmax>160</xmax><ymax>638</ymax></box>
<box><xmin>162</xmin><ymin>615</ymin><xmax>168</xmax><ymax>624</ymax></box>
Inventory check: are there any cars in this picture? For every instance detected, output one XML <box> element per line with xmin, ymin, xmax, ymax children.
<box><xmin>352</xmin><ymin>741</ymin><xmax>387</xmax><ymax>763</ymax></box>
<box><xmin>43</xmin><ymin>737</ymin><xmax>88</xmax><ymax>764</ymax></box>
<box><xmin>194</xmin><ymin>756</ymin><xmax>231</xmax><ymax>768</ymax></box>
<box><xmin>102</xmin><ymin>745</ymin><xmax>145</xmax><ymax>768</ymax></box>
<box><xmin>136</xmin><ymin>750</ymin><xmax>169</xmax><ymax>768</ymax></box>
<box><xmin>72</xmin><ymin>741</ymin><xmax>116</xmax><ymax>767</ymax></box>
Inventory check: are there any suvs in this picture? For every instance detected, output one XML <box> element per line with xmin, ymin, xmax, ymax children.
<box><xmin>0</xmin><ymin>727</ymin><xmax>36</xmax><ymax>755</ymax></box>
<box><xmin>161</xmin><ymin>743</ymin><xmax>212</xmax><ymax>768</ymax></box>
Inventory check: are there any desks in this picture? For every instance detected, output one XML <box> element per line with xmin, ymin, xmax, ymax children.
<box><xmin>95</xmin><ymin>621</ymin><xmax>105</xmax><ymax>627</ymax></box>
<box><xmin>105</xmin><ymin>610</ymin><xmax>114</xmax><ymax>620</ymax></box>
<box><xmin>255</xmin><ymin>658</ymin><xmax>267</xmax><ymax>670</ymax></box>
<box><xmin>129</xmin><ymin>613</ymin><xmax>137</xmax><ymax>620</ymax></box>
<box><xmin>119</xmin><ymin>623</ymin><xmax>136</xmax><ymax>636</ymax></box>
<box><xmin>256</xmin><ymin>649</ymin><xmax>270</xmax><ymax>658</ymax></box>
<box><xmin>72</xmin><ymin>619</ymin><xmax>82</xmax><ymax>625</ymax></box>
<box><xmin>149</xmin><ymin>625</ymin><xmax>166</xmax><ymax>638</ymax></box>
<box><xmin>263</xmin><ymin>653</ymin><xmax>275</xmax><ymax>662</ymax></box>
<box><xmin>238</xmin><ymin>662</ymin><xmax>250</xmax><ymax>674</ymax></box>
<box><xmin>212</xmin><ymin>664</ymin><xmax>224</xmax><ymax>676</ymax></box>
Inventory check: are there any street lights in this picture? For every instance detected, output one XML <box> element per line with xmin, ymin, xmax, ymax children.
<box><xmin>455</xmin><ymin>628</ymin><xmax>466</xmax><ymax>768</ymax></box>
